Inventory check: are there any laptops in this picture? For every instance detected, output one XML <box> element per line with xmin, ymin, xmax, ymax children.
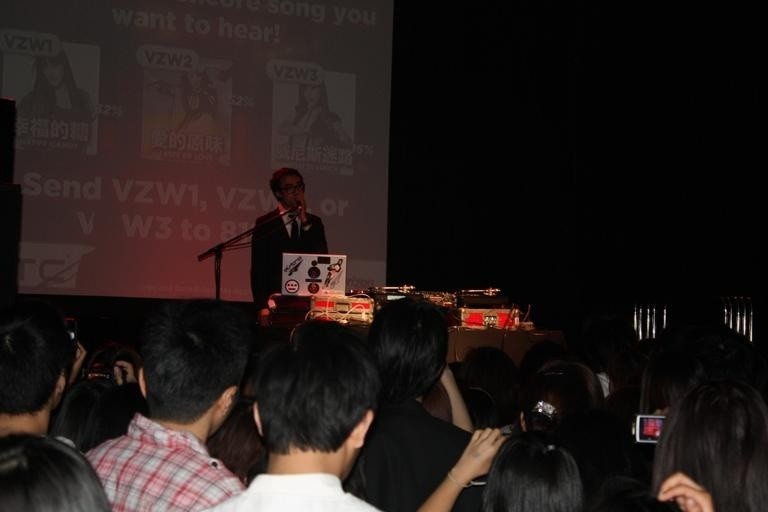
<box><xmin>280</xmin><ymin>252</ymin><xmax>349</xmax><ymax>296</ymax></box>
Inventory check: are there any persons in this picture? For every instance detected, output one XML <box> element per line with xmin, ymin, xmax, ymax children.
<box><xmin>1</xmin><ymin>295</ymin><xmax>768</xmax><ymax>512</ymax></box>
<box><xmin>150</xmin><ymin>63</ymin><xmax>233</xmax><ymax>166</ymax></box>
<box><xmin>250</xmin><ymin>167</ymin><xmax>331</xmax><ymax>301</ymax></box>
<box><xmin>282</xmin><ymin>68</ymin><xmax>346</xmax><ymax>168</ymax></box>
<box><xmin>18</xmin><ymin>47</ymin><xmax>94</xmax><ymax>151</ymax></box>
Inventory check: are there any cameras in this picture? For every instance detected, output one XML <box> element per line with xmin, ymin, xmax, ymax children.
<box><xmin>634</xmin><ymin>412</ymin><xmax>666</xmax><ymax>444</ymax></box>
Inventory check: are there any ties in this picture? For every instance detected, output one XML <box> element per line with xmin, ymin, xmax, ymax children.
<box><xmin>289</xmin><ymin>214</ymin><xmax>299</xmax><ymax>243</ymax></box>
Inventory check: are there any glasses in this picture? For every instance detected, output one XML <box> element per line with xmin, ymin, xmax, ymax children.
<box><xmin>277</xmin><ymin>183</ymin><xmax>305</xmax><ymax>195</ymax></box>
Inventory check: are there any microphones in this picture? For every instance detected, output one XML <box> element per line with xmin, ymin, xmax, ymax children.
<box><xmin>292</xmin><ymin>193</ymin><xmax>312</xmax><ymax>234</ymax></box>
